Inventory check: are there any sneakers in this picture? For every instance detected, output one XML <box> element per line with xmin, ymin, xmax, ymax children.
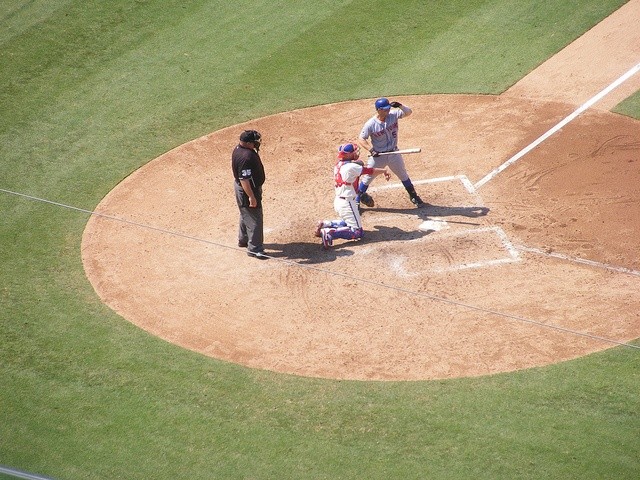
<box><xmin>315</xmin><ymin>221</ymin><xmax>326</xmax><ymax>237</ymax></box>
<box><xmin>357</xmin><ymin>203</ymin><xmax>360</xmax><ymax>214</ymax></box>
<box><xmin>410</xmin><ymin>195</ymin><xmax>423</xmax><ymax>208</ymax></box>
<box><xmin>248</xmin><ymin>250</ymin><xmax>267</xmax><ymax>259</ymax></box>
<box><xmin>320</xmin><ymin>228</ymin><xmax>329</xmax><ymax>250</ymax></box>
<box><xmin>238</xmin><ymin>241</ymin><xmax>247</xmax><ymax>246</ymax></box>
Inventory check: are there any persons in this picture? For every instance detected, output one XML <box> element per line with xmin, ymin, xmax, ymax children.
<box><xmin>355</xmin><ymin>98</ymin><xmax>424</xmax><ymax>214</ymax></box>
<box><xmin>315</xmin><ymin>143</ymin><xmax>391</xmax><ymax>250</ymax></box>
<box><xmin>232</xmin><ymin>130</ymin><xmax>268</xmax><ymax>260</ymax></box>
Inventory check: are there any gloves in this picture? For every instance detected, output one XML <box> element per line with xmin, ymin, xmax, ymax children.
<box><xmin>391</xmin><ymin>102</ymin><xmax>402</xmax><ymax>108</ymax></box>
<box><xmin>370</xmin><ymin>148</ymin><xmax>380</xmax><ymax>157</ymax></box>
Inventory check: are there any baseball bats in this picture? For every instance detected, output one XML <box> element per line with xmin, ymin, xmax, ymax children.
<box><xmin>368</xmin><ymin>148</ymin><xmax>421</xmax><ymax>157</ymax></box>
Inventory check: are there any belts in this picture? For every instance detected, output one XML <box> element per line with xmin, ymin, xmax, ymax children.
<box><xmin>379</xmin><ymin>146</ymin><xmax>397</xmax><ymax>153</ymax></box>
<box><xmin>235</xmin><ymin>180</ymin><xmax>241</xmax><ymax>186</ymax></box>
<box><xmin>340</xmin><ymin>197</ymin><xmax>345</xmax><ymax>200</ymax></box>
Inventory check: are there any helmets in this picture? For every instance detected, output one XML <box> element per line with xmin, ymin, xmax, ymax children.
<box><xmin>240</xmin><ymin>129</ymin><xmax>261</xmax><ymax>154</ymax></box>
<box><xmin>337</xmin><ymin>143</ymin><xmax>360</xmax><ymax>160</ymax></box>
<box><xmin>375</xmin><ymin>98</ymin><xmax>391</xmax><ymax>110</ymax></box>
